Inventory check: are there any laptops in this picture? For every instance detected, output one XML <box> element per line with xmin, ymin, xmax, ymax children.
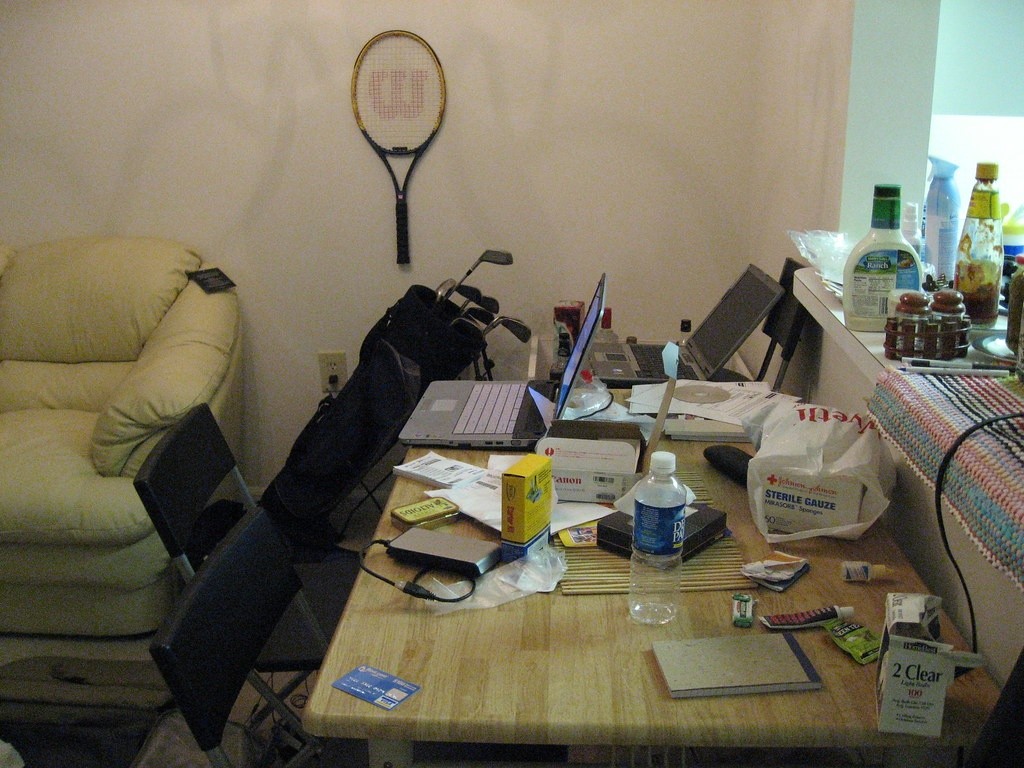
<box><xmin>398</xmin><ymin>273</ymin><xmax>608</xmax><ymax>448</ymax></box>
<box><xmin>589</xmin><ymin>264</ymin><xmax>786</xmax><ymax>389</ymax></box>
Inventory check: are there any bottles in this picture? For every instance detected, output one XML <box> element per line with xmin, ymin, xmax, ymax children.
<box><xmin>997</xmin><ymin>217</ymin><xmax>1024</xmax><ymax>314</ymax></box>
<box><xmin>592</xmin><ymin>306</ymin><xmax>620</xmax><ymax>345</ymax></box>
<box><xmin>843</xmin><ymin>184</ymin><xmax>924</xmax><ymax>333</ymax></box>
<box><xmin>929</xmin><ymin>289</ymin><xmax>966</xmax><ymax>360</ymax></box>
<box><xmin>627</xmin><ymin>450</ymin><xmax>687</xmax><ymax>625</ymax></box>
<box><xmin>669</xmin><ymin>319</ymin><xmax>694</xmax><ymax>346</ymax></box>
<box><xmin>893</xmin><ymin>292</ymin><xmax>932</xmax><ymax>360</ymax></box>
<box><xmin>900</xmin><ymin>201</ymin><xmax>922</xmax><ymax>262</ymax></box>
<box><xmin>951</xmin><ymin>161</ymin><xmax>1004</xmax><ymax>325</ymax></box>
<box><xmin>1007</xmin><ymin>254</ymin><xmax>1024</xmax><ymax>353</ymax></box>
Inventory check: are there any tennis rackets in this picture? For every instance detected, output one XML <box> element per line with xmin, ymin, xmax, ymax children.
<box><xmin>351</xmin><ymin>29</ymin><xmax>446</xmax><ymax>264</ymax></box>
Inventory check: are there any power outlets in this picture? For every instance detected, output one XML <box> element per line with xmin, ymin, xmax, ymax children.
<box><xmin>318</xmin><ymin>349</ymin><xmax>348</xmax><ymax>393</ymax></box>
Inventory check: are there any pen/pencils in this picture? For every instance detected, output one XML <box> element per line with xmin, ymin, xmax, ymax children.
<box><xmin>909</xmin><ymin>358</ymin><xmax>1016</xmax><ymax>371</ymax></box>
<box><xmin>897</xmin><ymin>365</ymin><xmax>1013</xmax><ymax>377</ymax></box>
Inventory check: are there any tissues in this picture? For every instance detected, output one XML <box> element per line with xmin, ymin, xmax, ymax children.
<box><xmin>596</xmin><ymin>479</ymin><xmax>727</xmax><ymax>572</ymax></box>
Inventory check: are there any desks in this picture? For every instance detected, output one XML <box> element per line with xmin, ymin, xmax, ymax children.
<box><xmin>303</xmin><ymin>388</ymin><xmax>999</xmax><ymax>768</ymax></box>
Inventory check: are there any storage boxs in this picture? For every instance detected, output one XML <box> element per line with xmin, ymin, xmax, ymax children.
<box><xmin>763</xmin><ymin>468</ymin><xmax>864</xmax><ymax>539</ymax></box>
<box><xmin>535</xmin><ymin>420</ymin><xmax>648</xmax><ymax>504</ymax></box>
<box><xmin>501</xmin><ymin>452</ymin><xmax>552</xmax><ymax>561</ymax></box>
<box><xmin>874</xmin><ymin>592</ymin><xmax>988</xmax><ymax>739</ymax></box>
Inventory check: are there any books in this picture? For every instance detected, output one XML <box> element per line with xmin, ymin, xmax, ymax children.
<box><xmin>652</xmin><ymin>633</ymin><xmax>821</xmax><ymax>699</ymax></box>
<box><xmin>663</xmin><ymin>381</ymin><xmax>773</xmax><ymax>442</ymax></box>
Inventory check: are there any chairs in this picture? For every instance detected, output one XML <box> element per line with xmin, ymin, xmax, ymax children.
<box><xmin>133</xmin><ymin>402</ymin><xmax>365</xmax><ymax>768</ymax></box>
<box><xmin>147</xmin><ymin>507</ymin><xmax>329</xmax><ymax>768</ymax></box>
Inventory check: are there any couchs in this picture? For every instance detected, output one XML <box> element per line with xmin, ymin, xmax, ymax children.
<box><xmin>0</xmin><ymin>235</ymin><xmax>248</xmax><ymax>723</ymax></box>
